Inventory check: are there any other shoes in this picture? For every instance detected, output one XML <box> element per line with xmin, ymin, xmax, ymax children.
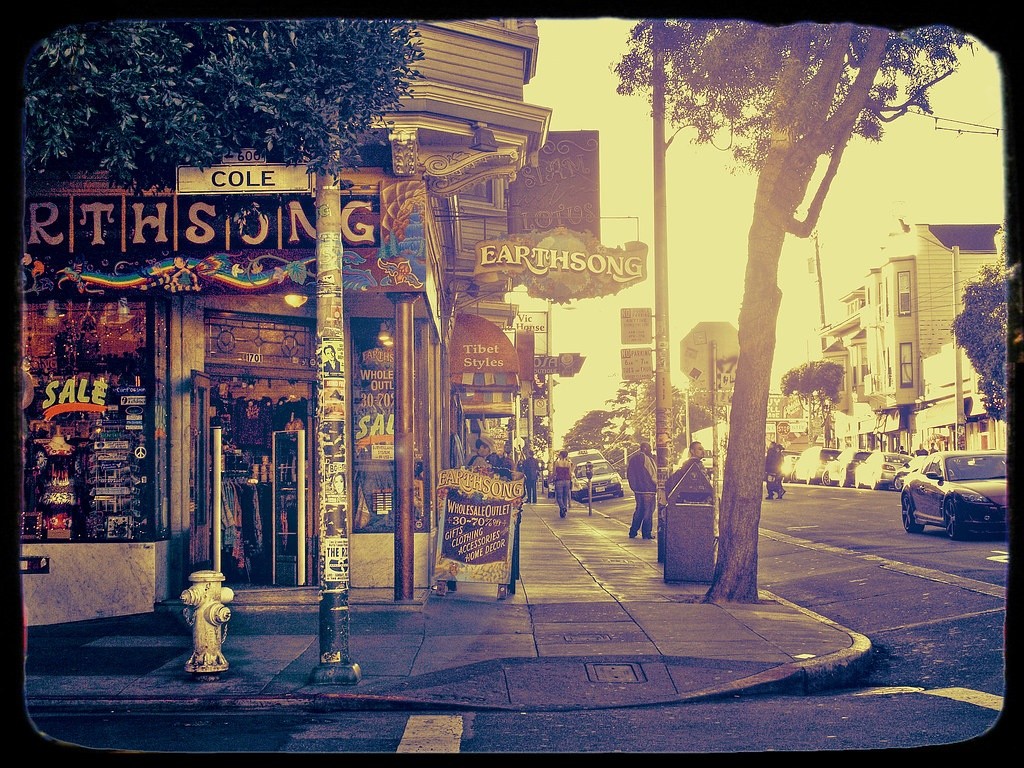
<box><xmin>781</xmin><ymin>489</ymin><xmax>786</xmax><ymax>495</ymax></box>
<box><xmin>776</xmin><ymin>497</ymin><xmax>782</xmax><ymax>499</ymax></box>
<box><xmin>643</xmin><ymin>536</ymin><xmax>655</xmax><ymax>539</ymax></box>
<box><xmin>766</xmin><ymin>495</ymin><xmax>773</xmax><ymax>499</ymax></box>
<box><xmin>561</xmin><ymin>508</ymin><xmax>566</xmax><ymax>518</ymax></box>
<box><xmin>524</xmin><ymin>499</ymin><xmax>531</xmax><ymax>503</ymax></box>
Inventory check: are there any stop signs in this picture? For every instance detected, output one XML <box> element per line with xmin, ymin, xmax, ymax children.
<box><xmin>776</xmin><ymin>421</ymin><xmax>791</xmax><ymax>436</ymax></box>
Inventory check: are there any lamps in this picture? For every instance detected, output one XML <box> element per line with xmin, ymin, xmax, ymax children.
<box><xmin>378</xmin><ymin>319</ymin><xmax>393</xmax><ymax>345</ymax></box>
<box><xmin>466</xmin><ymin>281</ymin><xmax>480</xmax><ymax>297</ymax></box>
<box><xmin>284</xmin><ymin>293</ymin><xmax>308</xmax><ymax>308</ymax></box>
<box><xmin>469</xmin><ymin>121</ymin><xmax>498</xmax><ymax>152</ymax></box>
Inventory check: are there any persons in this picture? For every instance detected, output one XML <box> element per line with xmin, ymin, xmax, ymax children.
<box><xmin>764</xmin><ymin>441</ymin><xmax>787</xmax><ymax>499</ymax></box>
<box><xmin>682</xmin><ymin>441</ymin><xmax>707</xmax><ymax>468</ymax></box>
<box><xmin>930</xmin><ymin>443</ymin><xmax>938</xmax><ymax>455</ymax></box>
<box><xmin>517</xmin><ymin>450</ymin><xmax>545</xmax><ymax>504</ymax></box>
<box><xmin>351</xmin><ymin>393</ymin><xmax>394</xmax><ymax>528</ymax></box>
<box><xmin>332</xmin><ymin>472</ymin><xmax>347</xmax><ymax>499</ymax></box>
<box><xmin>30</xmin><ymin>433</ymin><xmax>83</xmax><ymax>531</ymax></box>
<box><xmin>546</xmin><ymin>450</ymin><xmax>575</xmax><ymax>519</ymax></box>
<box><xmin>463</xmin><ymin>435</ymin><xmax>495</xmax><ymax>473</ymax></box>
<box><xmin>323</xmin><ymin>345</ymin><xmax>340</xmax><ymax>374</ymax></box>
<box><xmin>899</xmin><ymin>446</ymin><xmax>909</xmax><ymax>455</ymax></box>
<box><xmin>497</xmin><ymin>449</ymin><xmax>515</xmax><ymax>471</ymax></box>
<box><xmin>916</xmin><ymin>444</ymin><xmax>928</xmax><ymax>456</ymax></box>
<box><xmin>626</xmin><ymin>442</ymin><xmax>657</xmax><ymax>539</ymax></box>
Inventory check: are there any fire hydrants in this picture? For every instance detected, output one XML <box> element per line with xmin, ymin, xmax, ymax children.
<box><xmin>179</xmin><ymin>569</ymin><xmax>235</xmax><ymax>682</ymax></box>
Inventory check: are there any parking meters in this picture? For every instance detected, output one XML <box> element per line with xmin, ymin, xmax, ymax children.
<box><xmin>585</xmin><ymin>461</ymin><xmax>594</xmax><ymax>517</ymax></box>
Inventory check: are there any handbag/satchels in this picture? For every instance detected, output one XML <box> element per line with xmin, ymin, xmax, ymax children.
<box><xmin>768</xmin><ymin>472</ymin><xmax>776</xmax><ymax>482</ymax></box>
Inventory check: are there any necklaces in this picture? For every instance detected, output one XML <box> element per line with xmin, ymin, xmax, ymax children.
<box><xmin>51</xmin><ymin>440</ymin><xmax>66</xmax><ymax>448</ymax></box>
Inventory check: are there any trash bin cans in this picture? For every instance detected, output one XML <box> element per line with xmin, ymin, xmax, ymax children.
<box><xmin>664</xmin><ymin>461</ymin><xmax>716</xmax><ymax>584</ymax></box>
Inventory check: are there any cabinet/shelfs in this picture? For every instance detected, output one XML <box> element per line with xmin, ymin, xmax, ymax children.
<box><xmin>91</xmin><ymin>424</ymin><xmax>135</xmax><ymax>515</ymax></box>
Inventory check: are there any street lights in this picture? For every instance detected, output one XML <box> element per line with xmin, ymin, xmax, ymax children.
<box><xmin>897</xmin><ymin>218</ymin><xmax>966</xmax><ymax>449</ymax></box>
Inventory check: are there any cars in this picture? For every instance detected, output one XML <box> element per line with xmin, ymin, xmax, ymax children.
<box><xmin>762</xmin><ymin>448</ymin><xmax>937</xmax><ymax>493</ymax></box>
<box><xmin>570</xmin><ymin>458</ymin><xmax>624</xmax><ymax>503</ymax></box>
<box><xmin>899</xmin><ymin>449</ymin><xmax>1009</xmax><ymax>543</ymax></box>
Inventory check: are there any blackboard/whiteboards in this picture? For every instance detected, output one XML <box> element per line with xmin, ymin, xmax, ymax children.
<box><xmin>436</xmin><ymin>469</ymin><xmax>520</xmax><ymax>583</ymax></box>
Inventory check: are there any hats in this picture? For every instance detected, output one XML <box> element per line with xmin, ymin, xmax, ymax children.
<box><xmin>480</xmin><ymin>435</ymin><xmax>496</xmax><ymax>452</ymax></box>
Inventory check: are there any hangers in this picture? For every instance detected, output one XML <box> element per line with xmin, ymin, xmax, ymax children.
<box><xmin>244</xmin><ymin>382</ymin><xmax>301</xmax><ymax>402</ymax></box>
<box><xmin>222</xmin><ymin>469</ymin><xmax>253</xmax><ymax>490</ymax></box>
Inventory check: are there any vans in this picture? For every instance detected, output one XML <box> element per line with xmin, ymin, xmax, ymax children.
<box><xmin>553</xmin><ymin>447</ymin><xmax>615</xmax><ymax>479</ymax></box>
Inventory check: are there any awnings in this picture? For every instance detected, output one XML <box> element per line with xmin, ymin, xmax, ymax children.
<box><xmin>509</xmin><ymin>131</ymin><xmax>601</xmax><ymax>247</ymax></box>
<box><xmin>451</xmin><ymin>313</ymin><xmax>520</xmax><ymax>418</ymax></box>
